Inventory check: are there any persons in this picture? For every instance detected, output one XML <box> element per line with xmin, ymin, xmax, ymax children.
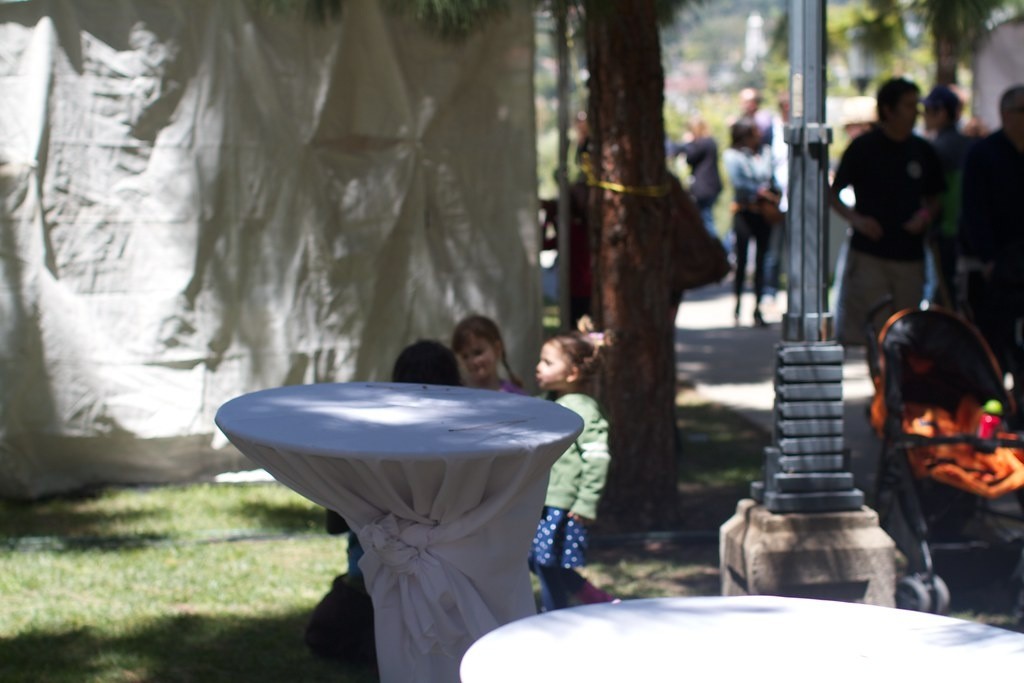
<box><xmin>829</xmin><ymin>76</ymin><xmax>945</xmax><ymax>411</ymax></box>
<box><xmin>452</xmin><ymin>317</ymin><xmax>526</xmax><ymax>396</ymax></box>
<box><xmin>304</xmin><ymin>338</ymin><xmax>462</xmax><ymax>667</ymax></box>
<box><xmin>661</xmin><ymin>86</ymin><xmax>790</xmax><ymax>327</ymax></box>
<box><xmin>529</xmin><ymin>317</ymin><xmax>622</xmax><ymax>614</ymax></box>
<box><xmin>917</xmin><ymin>85</ymin><xmax>1024</xmax><ymax>415</ymax></box>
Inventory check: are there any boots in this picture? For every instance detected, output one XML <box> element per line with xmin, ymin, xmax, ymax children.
<box><xmin>578</xmin><ymin>579</ymin><xmax>619</xmax><ymax>604</ymax></box>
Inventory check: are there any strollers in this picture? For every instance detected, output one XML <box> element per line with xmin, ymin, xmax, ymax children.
<box><xmin>861</xmin><ymin>290</ymin><xmax>1023</xmax><ymax>614</ymax></box>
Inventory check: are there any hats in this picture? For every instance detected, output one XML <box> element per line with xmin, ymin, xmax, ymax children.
<box><xmin>919</xmin><ymin>85</ymin><xmax>960</xmax><ymax>114</ymax></box>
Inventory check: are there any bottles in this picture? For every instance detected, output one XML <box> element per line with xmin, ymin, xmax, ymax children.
<box><xmin>974</xmin><ymin>398</ymin><xmax>1004</xmax><ymax>440</ymax></box>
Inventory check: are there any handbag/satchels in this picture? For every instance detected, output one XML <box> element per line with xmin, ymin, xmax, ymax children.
<box><xmin>760</xmin><ymin>187</ymin><xmax>783</xmax><ymax>224</ymax></box>
